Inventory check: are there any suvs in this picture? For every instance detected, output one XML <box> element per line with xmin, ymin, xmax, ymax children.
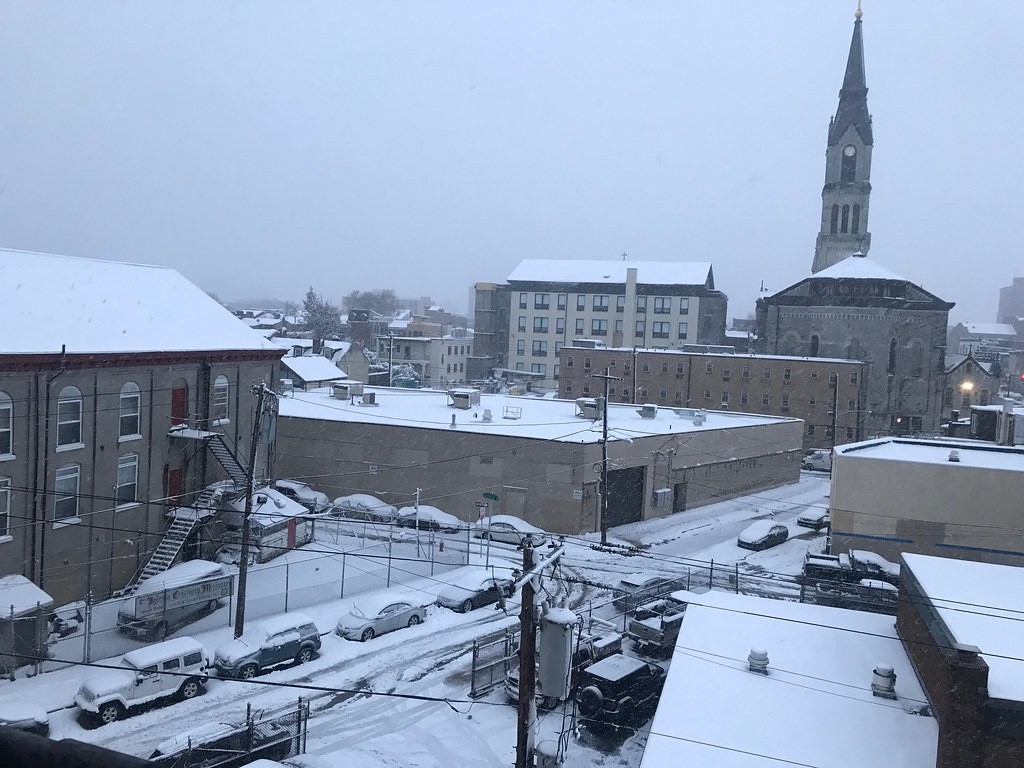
<box><xmin>74</xmin><ymin>637</ymin><xmax>209</xmax><ymax>724</ymax></box>
<box><xmin>574</xmin><ymin>652</ymin><xmax>667</xmax><ymax>731</ymax></box>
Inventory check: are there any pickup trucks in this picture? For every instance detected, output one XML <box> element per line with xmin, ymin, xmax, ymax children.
<box><xmin>628</xmin><ymin>590</ymin><xmax>700</xmax><ymax>652</ymax></box>
<box><xmin>504</xmin><ymin>633</ymin><xmax>623</xmax><ymax>709</ymax></box>
<box><xmin>803</xmin><ymin>549</ymin><xmax>900</xmax><ymax>587</ymax></box>
<box><xmin>816</xmin><ymin>579</ymin><xmax>899</xmax><ymax>615</ymax></box>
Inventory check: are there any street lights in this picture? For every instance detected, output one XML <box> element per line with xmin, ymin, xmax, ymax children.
<box><xmin>593</xmin><ymin>367</ymin><xmax>633</xmax><ymax>547</ymax></box>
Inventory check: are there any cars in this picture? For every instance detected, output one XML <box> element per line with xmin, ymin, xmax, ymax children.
<box><xmin>475</xmin><ymin>514</ymin><xmax>546</xmax><ymax>547</ymax></box>
<box><xmin>611</xmin><ymin>573</ymin><xmax>686</xmax><ymax>615</ymax></box>
<box><xmin>0</xmin><ymin>706</ymin><xmax>49</xmax><ymax>737</ymax></box>
<box><xmin>148</xmin><ymin>719</ymin><xmax>292</xmax><ymax>768</ymax></box>
<box><xmin>336</xmin><ymin>593</ymin><xmax>425</xmax><ymax>642</ymax></box>
<box><xmin>333</xmin><ymin>494</ymin><xmax>398</xmax><ymax>522</ymax></box>
<box><xmin>438</xmin><ymin>570</ymin><xmax>514</xmax><ymax>613</ymax></box>
<box><xmin>213</xmin><ymin>615</ymin><xmax>321</xmax><ymax>680</ymax></box>
<box><xmin>797</xmin><ymin>504</ymin><xmax>830</xmax><ymax>529</ymax></box>
<box><xmin>266</xmin><ymin>480</ymin><xmax>329</xmax><ymax>514</ymax></box>
<box><xmin>397</xmin><ymin>505</ymin><xmax>460</xmax><ymax>534</ymax></box>
<box><xmin>737</xmin><ymin>519</ymin><xmax>789</xmax><ymax>552</ymax></box>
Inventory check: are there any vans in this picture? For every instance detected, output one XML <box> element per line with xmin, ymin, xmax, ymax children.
<box><xmin>116</xmin><ymin>559</ymin><xmax>225</xmax><ymax>641</ymax></box>
<box><xmin>801</xmin><ymin>451</ymin><xmax>832</xmax><ymax>471</ymax></box>
